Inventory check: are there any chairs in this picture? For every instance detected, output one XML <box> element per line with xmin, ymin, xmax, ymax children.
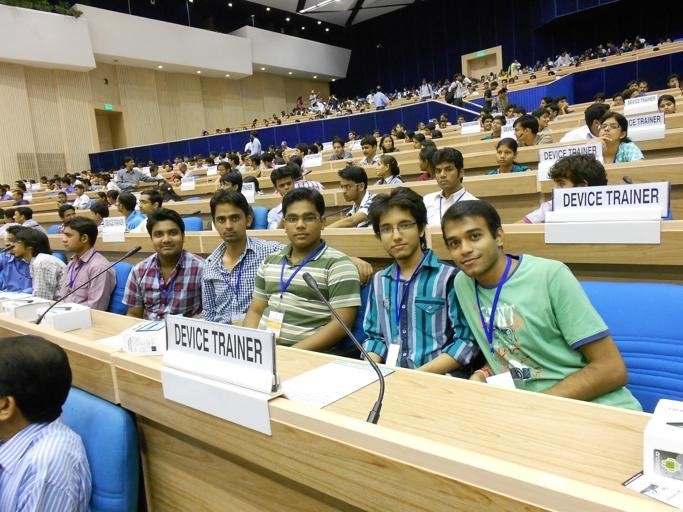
<box><xmin>0</xmin><ymin>386</ymin><xmax>140</xmax><ymax>512</ymax></box>
<box><xmin>216</xmin><ymin>40</ymin><xmax>682</xmax><ymax>135</ymax></box>
<box><xmin>1</xmin><ymin>136</ymin><xmax>683</xmax><ymax>413</ymax></box>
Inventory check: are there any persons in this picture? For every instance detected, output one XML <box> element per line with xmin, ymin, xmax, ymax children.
<box><xmin>12</xmin><ymin>227</ymin><xmax>67</xmax><ymax>302</ymax></box>
<box><xmin>199</xmin><ymin>187</ymin><xmax>376</xmax><ymax>325</ymax></box>
<box><xmin>241</xmin><ymin>188</ymin><xmax>361</xmax><ymax>353</ymax></box>
<box><xmin>442</xmin><ymin>201</ymin><xmax>644</xmax><ymax>412</ymax></box>
<box><xmin>0</xmin><ymin>226</ymin><xmax>34</xmax><ymax>293</ymax></box>
<box><xmin>358</xmin><ymin>184</ymin><xmax>480</xmax><ymax>379</ymax></box>
<box><xmin>1</xmin><ymin>334</ymin><xmax>94</xmax><ymax>512</ymax></box>
<box><xmin>57</xmin><ymin>216</ymin><xmax>118</xmax><ymax>313</ymax></box>
<box><xmin>120</xmin><ymin>209</ymin><xmax>207</xmax><ymax>321</ymax></box>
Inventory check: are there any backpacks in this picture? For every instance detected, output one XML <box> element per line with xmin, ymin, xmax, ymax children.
<box><xmin>445</xmin><ymin>81</ymin><xmax>458</xmax><ymax>105</ymax></box>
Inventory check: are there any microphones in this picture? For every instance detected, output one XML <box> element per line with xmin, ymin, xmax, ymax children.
<box><xmin>296</xmin><ymin>170</ymin><xmax>312</xmax><ymax>181</ymax></box>
<box><xmin>32</xmin><ymin>245</ymin><xmax>142</xmax><ymax>325</ymax></box>
<box><xmin>516</xmin><ymin>133</ymin><xmax>524</xmax><ymax>141</ymax></box>
<box><xmin>622</xmin><ymin>176</ymin><xmax>633</xmax><ymax>184</ymax></box>
<box><xmin>302</xmin><ymin>271</ymin><xmax>385</xmax><ymax>424</ymax></box>
<box><xmin>181</xmin><ymin>210</ymin><xmax>202</xmax><ymax>218</ymax></box>
<box><xmin>0</xmin><ymin>243</ymin><xmax>16</xmax><ymax>257</ymax></box>
<box><xmin>321</xmin><ymin>207</ymin><xmax>350</xmax><ymax>219</ymax></box>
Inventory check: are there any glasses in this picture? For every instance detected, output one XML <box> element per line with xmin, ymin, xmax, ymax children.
<box><xmin>598</xmin><ymin>123</ymin><xmax>622</xmax><ymax>131</ymax></box>
<box><xmin>340</xmin><ymin>183</ymin><xmax>361</xmax><ymax>190</ymax></box>
<box><xmin>376</xmin><ymin>220</ymin><xmax>419</xmax><ymax>234</ymax></box>
<box><xmin>282</xmin><ymin>213</ymin><xmax>322</xmax><ymax>223</ymax></box>
